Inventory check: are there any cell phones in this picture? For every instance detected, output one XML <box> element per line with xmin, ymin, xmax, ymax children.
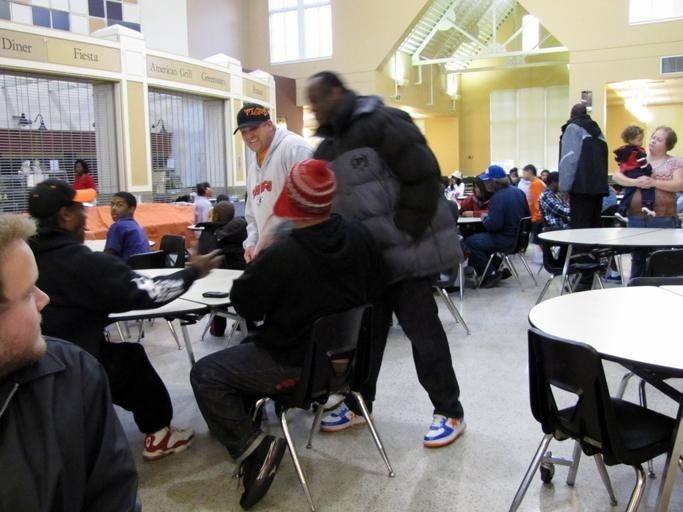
<box><xmin>202</xmin><ymin>290</ymin><xmax>230</xmax><ymax>298</ymax></box>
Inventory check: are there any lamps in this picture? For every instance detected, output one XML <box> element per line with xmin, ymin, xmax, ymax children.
<box><xmin>521</xmin><ymin>15</ymin><xmax>540</xmax><ymax>51</ymax></box>
<box><xmin>391</xmin><ymin>54</ymin><xmax>407</xmax><ymax>87</ymax></box>
<box><xmin>446</xmin><ymin>74</ymin><xmax>460</xmax><ymax>99</ymax></box>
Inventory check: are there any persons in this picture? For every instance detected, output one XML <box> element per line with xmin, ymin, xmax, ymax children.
<box><xmin>0</xmin><ymin>211</ymin><xmax>138</xmax><ymax>512</ymax></box>
<box><xmin>207</xmin><ymin>193</ymin><xmax>230</xmax><ymax>222</ymax></box>
<box><xmin>460</xmin><ymin>173</ymin><xmax>494</xmax><ymax>217</ymax></box>
<box><xmin>443</xmin><ymin>170</ymin><xmax>465</xmax><ymax>201</ymax></box>
<box><xmin>305</xmin><ymin>70</ymin><xmax>468</xmax><ymax>448</ymax></box>
<box><xmin>103</xmin><ymin>192</ymin><xmax>151</xmax><ymax>261</ymax></box>
<box><xmin>522</xmin><ymin>164</ymin><xmax>547</xmax><ymax>231</ymax></box>
<box><xmin>537</xmin><ymin>171</ymin><xmax>595</xmax><ymax>293</ymax></box>
<box><xmin>612</xmin><ymin>125</ymin><xmax>683</xmax><ymax>281</ymax></box>
<box><xmin>192</xmin><ymin>182</ymin><xmax>213</xmax><ymax>223</ymax></box>
<box><xmin>188</xmin><ymin>157</ymin><xmax>377</xmax><ymax>511</ymax></box>
<box><xmin>232</xmin><ymin>102</ymin><xmax>348</xmax><ymax>411</ymax></box>
<box><xmin>558</xmin><ymin>103</ymin><xmax>622</xmax><ymax>228</ymax></box>
<box><xmin>540</xmin><ymin>169</ymin><xmax>550</xmax><ymax>183</ymax></box>
<box><xmin>197</xmin><ymin>200</ymin><xmax>247</xmax><ymax>269</ymax></box>
<box><xmin>507</xmin><ymin>168</ymin><xmax>521</xmax><ymax>187</ymax></box>
<box><xmin>26</xmin><ymin>178</ymin><xmax>225</xmax><ymax>463</ymax></box>
<box><xmin>71</xmin><ymin>158</ymin><xmax>98</xmax><ymax>205</ymax></box>
<box><xmin>465</xmin><ymin>165</ymin><xmax>530</xmax><ymax>287</ymax></box>
<box><xmin>613</xmin><ymin>125</ymin><xmax>657</xmax><ymax>223</ymax></box>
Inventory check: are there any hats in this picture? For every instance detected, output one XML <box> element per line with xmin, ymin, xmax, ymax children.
<box><xmin>479</xmin><ymin>165</ymin><xmax>507</xmax><ymax>182</ymax></box>
<box><xmin>450</xmin><ymin>170</ymin><xmax>462</xmax><ymax>180</ymax></box>
<box><xmin>232</xmin><ymin>103</ymin><xmax>271</xmax><ymax>136</ymax></box>
<box><xmin>273</xmin><ymin>158</ymin><xmax>338</xmax><ymax>221</ymax></box>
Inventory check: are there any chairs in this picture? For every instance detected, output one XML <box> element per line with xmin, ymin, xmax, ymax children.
<box><xmin>510</xmin><ymin>327</ymin><xmax>683</xmax><ymax>511</ymax></box>
<box><xmin>129</xmin><ymin>249</ymin><xmax>165</xmax><ymax>272</ymax></box>
<box><xmin>432</xmin><ymin>261</ymin><xmax>472</xmax><ymax>336</ymax></box>
<box><xmin>138</xmin><ymin>233</ymin><xmax>185</xmax><ymax>351</ymax></box>
<box><xmin>231</xmin><ymin>303</ymin><xmax>396</xmax><ymax>511</ymax></box>
<box><xmin>475</xmin><ymin>216</ymin><xmax>538</xmax><ymax>292</ymax></box>
<box><xmin>644</xmin><ymin>248</ymin><xmax>683</xmax><ymax>277</ymax></box>
<box><xmin>533</xmin><ymin>241</ymin><xmax>608</xmax><ymax>305</ymax></box>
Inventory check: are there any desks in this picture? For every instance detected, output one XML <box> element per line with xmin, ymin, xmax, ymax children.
<box><xmin>535</xmin><ymin>225</ymin><xmax>683</xmax><ymax>296</ymax></box>
<box><xmin>527</xmin><ymin>284</ymin><xmax>683</xmax><ymax>512</ymax></box>
<box><xmin>103</xmin><ymin>266</ymin><xmax>247</xmax><ymax>321</ymax></box>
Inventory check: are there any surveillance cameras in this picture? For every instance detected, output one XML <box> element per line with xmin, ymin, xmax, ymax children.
<box><xmin>389</xmin><ymin>93</ymin><xmax>403</xmax><ymax>102</ymax></box>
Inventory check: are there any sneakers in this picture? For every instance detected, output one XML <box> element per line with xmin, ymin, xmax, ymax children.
<box><xmin>319</xmin><ymin>400</ymin><xmax>376</xmax><ymax>433</ymax></box>
<box><xmin>141</xmin><ymin>425</ymin><xmax>196</xmax><ymax>460</ymax></box>
<box><xmin>231</xmin><ymin>434</ymin><xmax>287</xmax><ymax>511</ymax></box>
<box><xmin>484</xmin><ymin>272</ymin><xmax>502</xmax><ymax>288</ymax></box>
<box><xmin>603</xmin><ymin>273</ymin><xmax>621</xmax><ymax>283</ymax></box>
<box><xmin>641</xmin><ymin>206</ymin><xmax>657</xmax><ymax>217</ymax></box>
<box><xmin>422</xmin><ymin>411</ymin><xmax>467</xmax><ymax>448</ymax></box>
<box><xmin>614</xmin><ymin>212</ymin><xmax>630</xmax><ymax>223</ymax></box>
<box><xmin>501</xmin><ymin>267</ymin><xmax>512</xmax><ymax>280</ymax></box>
